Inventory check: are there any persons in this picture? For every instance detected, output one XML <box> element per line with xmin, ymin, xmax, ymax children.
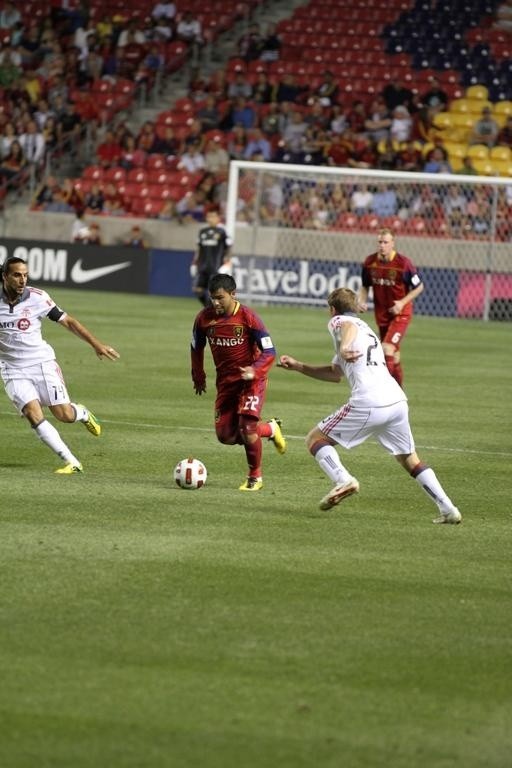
<box><xmin>190</xmin><ymin>273</ymin><xmax>285</xmax><ymax>490</ymax></box>
<box><xmin>281</xmin><ymin>69</ymin><xmax>512</xmax><ymax>231</ymax></box>
<box><xmin>189</xmin><ymin>207</ymin><xmax>232</xmax><ymax>306</ymax></box>
<box><xmin>275</xmin><ymin>287</ymin><xmax>463</xmax><ymax>523</ymax></box>
<box><xmin>0</xmin><ymin>256</ymin><xmax>120</xmax><ymax>472</ymax></box>
<box><xmin>358</xmin><ymin>227</ymin><xmax>424</xmax><ymax>387</ymax></box>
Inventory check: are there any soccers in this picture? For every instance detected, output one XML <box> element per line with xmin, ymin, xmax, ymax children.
<box><xmin>174</xmin><ymin>459</ymin><xmax>207</xmax><ymax>490</ymax></box>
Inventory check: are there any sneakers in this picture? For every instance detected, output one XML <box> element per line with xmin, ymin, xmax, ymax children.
<box><xmin>267</xmin><ymin>417</ymin><xmax>288</xmax><ymax>455</ymax></box>
<box><xmin>432</xmin><ymin>510</ymin><xmax>463</xmax><ymax>525</ymax></box>
<box><xmin>54</xmin><ymin>463</ymin><xmax>84</xmax><ymax>475</ymax></box>
<box><xmin>318</xmin><ymin>477</ymin><xmax>360</xmax><ymax>511</ymax></box>
<box><xmin>239</xmin><ymin>476</ymin><xmax>264</xmax><ymax>492</ymax></box>
<box><xmin>76</xmin><ymin>403</ymin><xmax>102</xmax><ymax>437</ymax></box>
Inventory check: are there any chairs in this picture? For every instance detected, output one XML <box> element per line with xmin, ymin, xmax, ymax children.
<box><xmin>1</xmin><ymin>2</ymin><xmax>511</xmax><ymax>256</ymax></box>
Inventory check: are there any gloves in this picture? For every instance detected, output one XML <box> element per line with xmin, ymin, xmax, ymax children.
<box><xmin>218</xmin><ymin>263</ymin><xmax>231</xmax><ymax>276</ymax></box>
<box><xmin>189</xmin><ymin>264</ymin><xmax>199</xmax><ymax>277</ymax></box>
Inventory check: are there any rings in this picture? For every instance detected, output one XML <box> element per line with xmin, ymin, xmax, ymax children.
<box><xmin>109</xmin><ymin>347</ymin><xmax>114</xmax><ymax>351</ymax></box>
